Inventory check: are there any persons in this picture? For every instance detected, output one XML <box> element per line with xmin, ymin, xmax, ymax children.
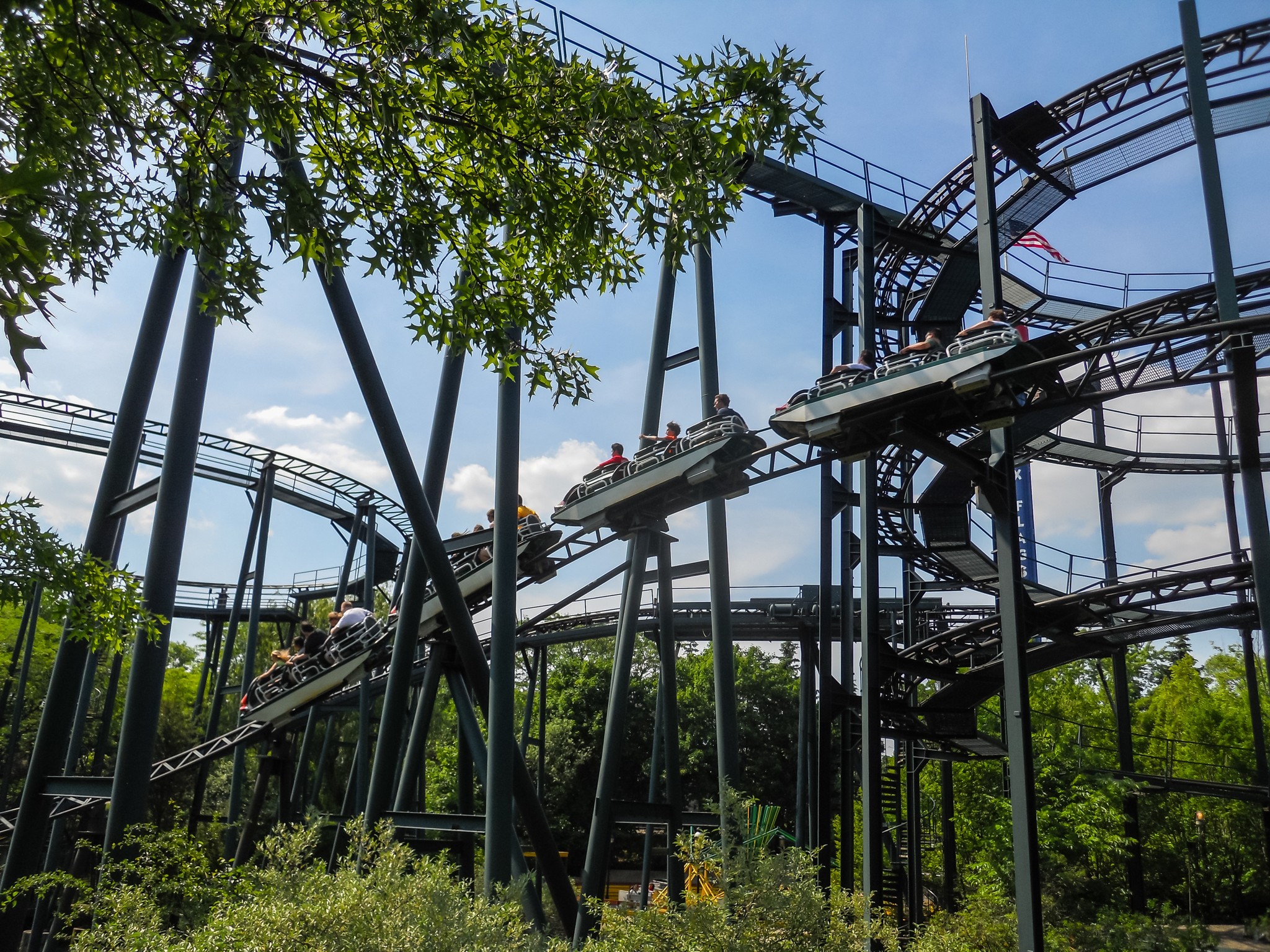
<box><xmin>956</xmin><ymin>310</ymin><xmax>1007</xmax><ymax>335</ymax></box>
<box><xmin>638</xmin><ymin>421</ymin><xmax>681</xmax><ymax>452</ymax></box>
<box><xmin>713</xmin><ymin>393</ymin><xmax>749</xmax><ymax>432</ymax></box>
<box><xmin>898</xmin><ymin>328</ymin><xmax>942</xmax><ymax>355</ymax></box>
<box><xmin>591</xmin><ymin>442</ymin><xmax>629</xmax><ymax>472</ymax></box>
<box><xmin>258</xmin><ymin>601</ymin><xmax>375</xmax><ymax>697</ymax></box>
<box><xmin>830</xmin><ymin>350</ymin><xmax>873</xmax><ymax>374</ymax></box>
<box><xmin>451</xmin><ymin>495</ymin><xmax>541</xmax><ymax>563</ymax></box>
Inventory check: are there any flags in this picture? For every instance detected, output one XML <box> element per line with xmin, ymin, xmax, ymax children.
<box><xmin>1010</xmin><ymin>219</ymin><xmax>1070</xmax><ymax>265</ymax></box>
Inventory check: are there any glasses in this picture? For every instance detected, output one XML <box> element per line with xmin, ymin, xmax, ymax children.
<box><xmin>329</xmin><ymin>618</ymin><xmax>333</xmax><ymax>621</ymax></box>
<box><xmin>925</xmin><ymin>333</ymin><xmax>932</xmax><ymax>338</ymax></box>
